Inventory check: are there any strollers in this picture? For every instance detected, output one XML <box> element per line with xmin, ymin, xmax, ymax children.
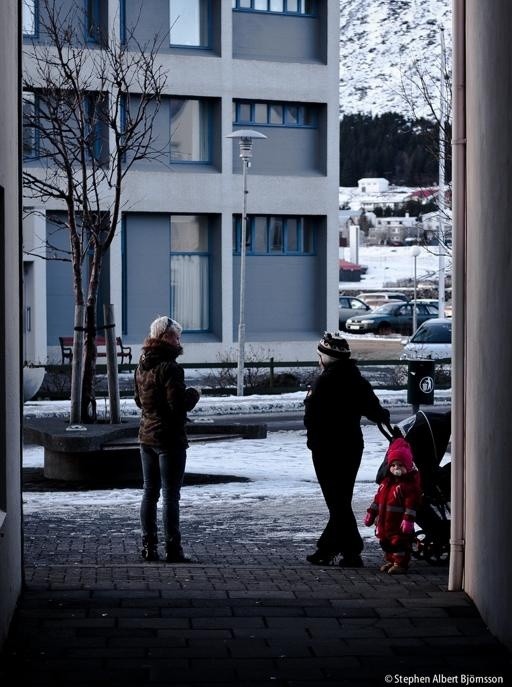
<box><xmin>375</xmin><ymin>406</ymin><xmax>451</xmax><ymax>566</ymax></box>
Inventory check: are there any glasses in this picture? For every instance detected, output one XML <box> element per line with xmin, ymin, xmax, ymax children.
<box><xmin>165</xmin><ymin>318</ymin><xmax>176</xmax><ymax>332</ymax></box>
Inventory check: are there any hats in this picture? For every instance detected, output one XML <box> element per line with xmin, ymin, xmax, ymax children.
<box><xmin>317</xmin><ymin>331</ymin><xmax>351</xmax><ymax>376</ymax></box>
<box><xmin>388</xmin><ymin>438</ymin><xmax>413</xmax><ymax>473</ymax></box>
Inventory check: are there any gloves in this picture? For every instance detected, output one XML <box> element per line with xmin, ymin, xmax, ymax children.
<box><xmin>400</xmin><ymin>520</ymin><xmax>414</xmax><ymax>534</ymax></box>
<box><xmin>365</xmin><ymin>513</ymin><xmax>375</xmax><ymax>526</ymax></box>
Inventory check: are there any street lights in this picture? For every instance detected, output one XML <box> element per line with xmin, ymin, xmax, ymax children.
<box><xmin>410</xmin><ymin>246</ymin><xmax>421</xmax><ymax>335</ymax></box>
<box><xmin>222</xmin><ymin>129</ymin><xmax>271</xmax><ymax>394</ymax></box>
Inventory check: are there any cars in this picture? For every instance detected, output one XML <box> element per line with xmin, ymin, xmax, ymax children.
<box><xmin>397</xmin><ymin>316</ymin><xmax>452</xmax><ymax>382</ymax></box>
<box><xmin>338</xmin><ymin>289</ymin><xmax>451</xmax><ymax>337</ymax></box>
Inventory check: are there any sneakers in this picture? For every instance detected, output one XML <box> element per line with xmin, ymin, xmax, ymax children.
<box><xmin>166</xmin><ymin>553</ymin><xmax>191</xmax><ymax>563</ymax></box>
<box><xmin>307</xmin><ymin>548</ymin><xmax>364</xmax><ymax>567</ymax></box>
<box><xmin>380</xmin><ymin>562</ymin><xmax>408</xmax><ymax>574</ymax></box>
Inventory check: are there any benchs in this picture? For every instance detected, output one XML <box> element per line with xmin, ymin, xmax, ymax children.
<box><xmin>58</xmin><ymin>333</ymin><xmax>133</xmax><ymax>372</ymax></box>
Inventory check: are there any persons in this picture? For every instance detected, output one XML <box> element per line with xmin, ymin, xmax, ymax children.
<box><xmin>364</xmin><ymin>437</ymin><xmax>421</xmax><ymax>575</ymax></box>
<box><xmin>134</xmin><ymin>316</ymin><xmax>203</xmax><ymax>563</ymax></box>
<box><xmin>304</xmin><ymin>330</ymin><xmax>390</xmax><ymax>567</ymax></box>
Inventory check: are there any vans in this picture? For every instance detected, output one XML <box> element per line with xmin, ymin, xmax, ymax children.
<box><xmin>367</xmin><ymin>223</ymin><xmax>447</xmax><ymax>246</ymax></box>
